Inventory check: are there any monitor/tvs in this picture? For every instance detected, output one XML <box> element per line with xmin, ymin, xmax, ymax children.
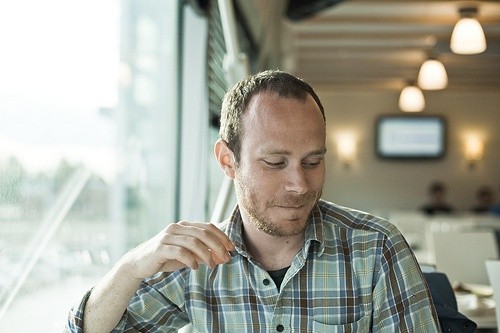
<box><xmin>374</xmin><ymin>114</ymin><xmax>446</xmax><ymax>159</ymax></box>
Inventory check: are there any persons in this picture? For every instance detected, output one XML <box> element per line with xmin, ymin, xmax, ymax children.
<box><xmin>68</xmin><ymin>69</ymin><xmax>442</xmax><ymax>333</ymax></box>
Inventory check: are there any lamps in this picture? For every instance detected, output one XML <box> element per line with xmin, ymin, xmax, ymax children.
<box><xmin>399</xmin><ymin>80</ymin><xmax>425</xmax><ymax>112</ymax></box>
<box><xmin>418</xmin><ymin>55</ymin><xmax>448</xmax><ymax>90</ymax></box>
<box><xmin>450</xmin><ymin>8</ymin><xmax>487</xmax><ymax>55</ymax></box>
